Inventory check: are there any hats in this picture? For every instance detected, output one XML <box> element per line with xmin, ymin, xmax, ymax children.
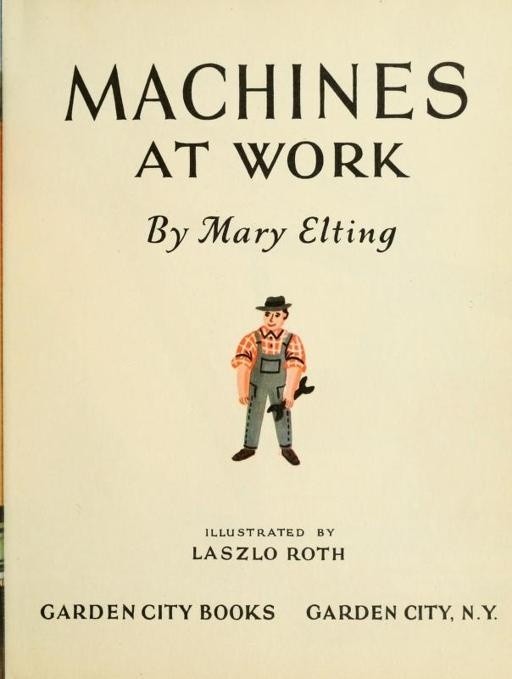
<box><xmin>254</xmin><ymin>296</ymin><xmax>293</xmax><ymax>313</ymax></box>
